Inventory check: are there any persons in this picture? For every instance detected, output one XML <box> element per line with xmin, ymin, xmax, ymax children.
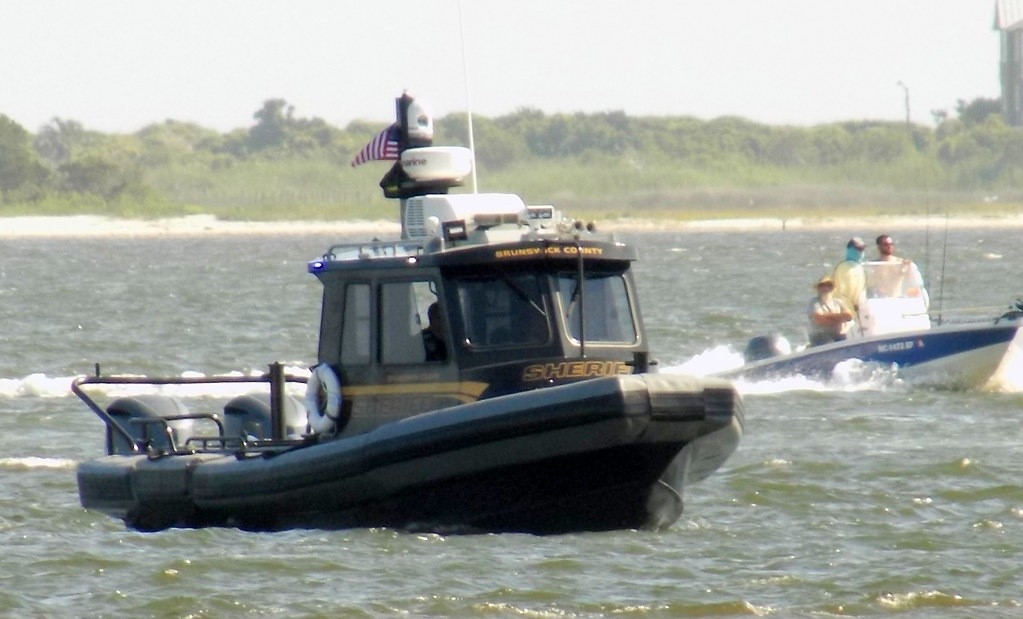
<box><xmin>806</xmin><ymin>234</ymin><xmax>905</xmax><ymax>347</ymax></box>
<box><xmin>420</xmin><ymin>302</ymin><xmax>448</xmax><ymax>364</ymax></box>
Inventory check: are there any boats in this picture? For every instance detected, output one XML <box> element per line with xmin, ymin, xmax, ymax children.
<box><xmin>69</xmin><ymin>86</ymin><xmax>745</xmax><ymax>536</ymax></box>
<box><xmin>706</xmin><ymin>203</ymin><xmax>1022</xmax><ymax>393</ymax></box>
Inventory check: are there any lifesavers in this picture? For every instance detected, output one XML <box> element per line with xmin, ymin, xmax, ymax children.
<box><xmin>305</xmin><ymin>364</ymin><xmax>342</xmax><ymax>433</ymax></box>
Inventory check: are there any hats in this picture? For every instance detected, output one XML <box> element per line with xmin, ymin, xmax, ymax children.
<box><xmin>847</xmin><ymin>237</ymin><xmax>868</xmax><ymax>248</ymax></box>
<box><xmin>819</xmin><ymin>276</ymin><xmax>833</xmax><ymax>285</ymax></box>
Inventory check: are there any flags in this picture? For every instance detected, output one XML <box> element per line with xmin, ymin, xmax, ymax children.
<box><xmin>350</xmin><ymin>122</ymin><xmax>401</xmax><ymax>168</ymax></box>
